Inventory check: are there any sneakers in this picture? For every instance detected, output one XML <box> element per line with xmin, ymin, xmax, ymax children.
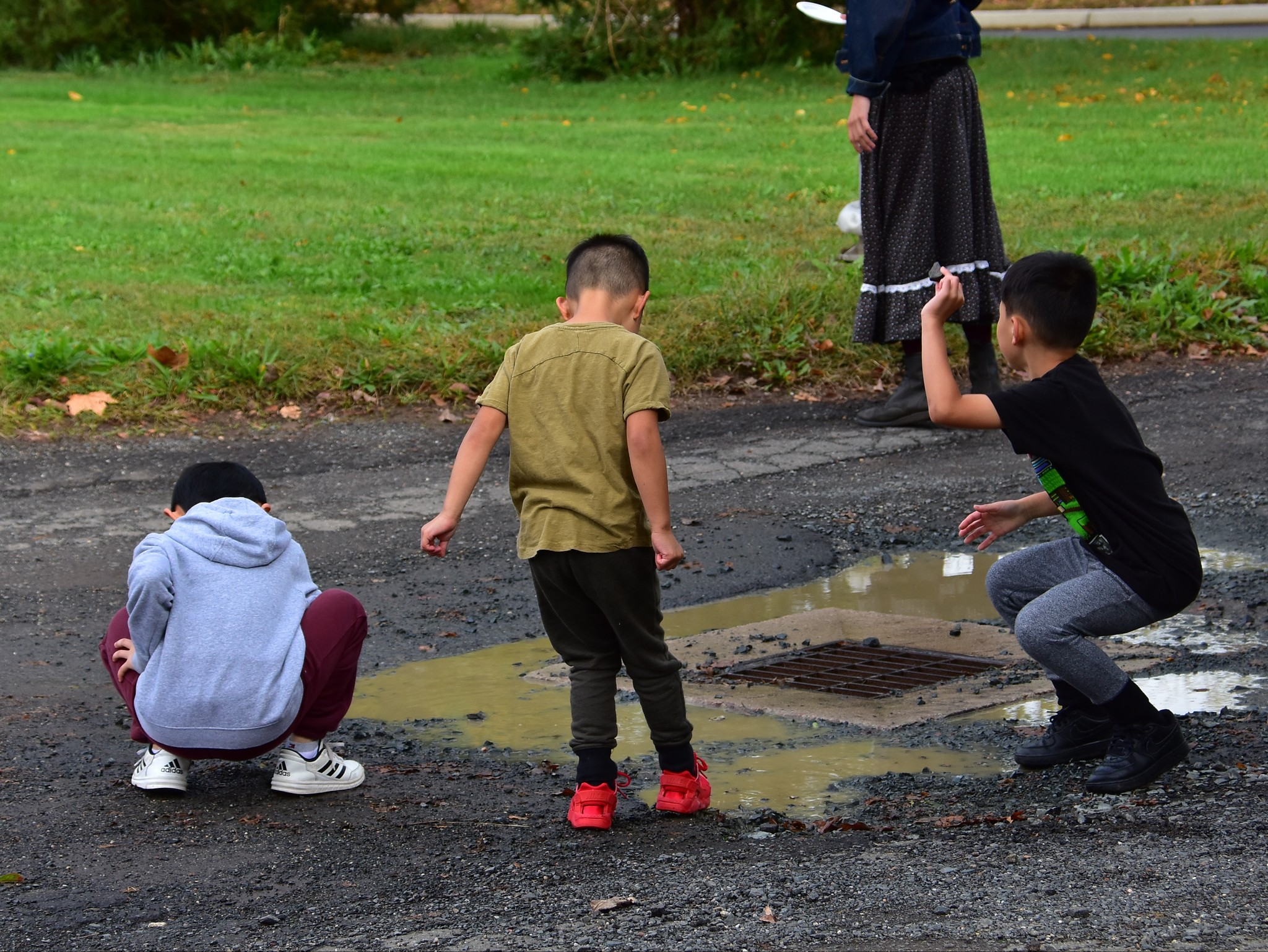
<box><xmin>270</xmin><ymin>740</ymin><xmax>365</xmax><ymax>795</ymax></box>
<box><xmin>566</xmin><ymin>771</ymin><xmax>630</xmax><ymax>830</ymax></box>
<box><xmin>655</xmin><ymin>750</ymin><xmax>712</xmax><ymax>815</ymax></box>
<box><xmin>131</xmin><ymin>743</ymin><xmax>190</xmax><ymax>792</ymax></box>
<box><xmin>1014</xmin><ymin>705</ymin><xmax>1116</xmax><ymax>766</ymax></box>
<box><xmin>1086</xmin><ymin>708</ymin><xmax>1189</xmax><ymax>794</ymax></box>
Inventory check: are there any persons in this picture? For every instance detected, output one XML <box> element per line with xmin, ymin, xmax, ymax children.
<box><xmin>833</xmin><ymin>0</ymin><xmax>1012</xmax><ymax>427</ymax></box>
<box><xmin>920</xmin><ymin>251</ymin><xmax>1204</xmax><ymax>793</ymax></box>
<box><xmin>99</xmin><ymin>460</ymin><xmax>368</xmax><ymax>795</ymax></box>
<box><xmin>420</xmin><ymin>234</ymin><xmax>711</xmax><ymax>827</ymax></box>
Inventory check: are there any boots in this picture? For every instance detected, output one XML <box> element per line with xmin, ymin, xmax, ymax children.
<box><xmin>965</xmin><ymin>342</ymin><xmax>1003</xmax><ymax>396</ymax></box>
<box><xmin>854</xmin><ymin>347</ymin><xmax>930</xmax><ymax>428</ymax></box>
